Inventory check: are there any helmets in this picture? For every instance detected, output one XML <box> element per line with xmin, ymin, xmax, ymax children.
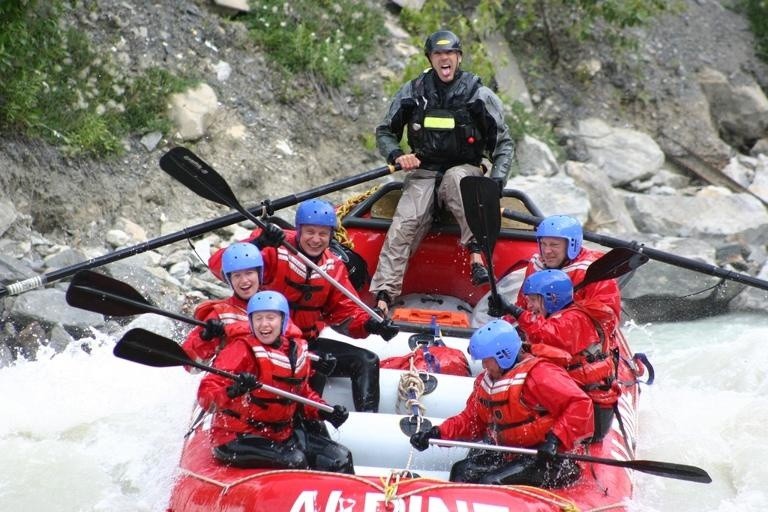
<box><xmin>536</xmin><ymin>215</ymin><xmax>584</xmax><ymax>261</ymax></box>
<box><xmin>521</xmin><ymin>269</ymin><xmax>574</xmax><ymax>316</ymax></box>
<box><xmin>424</xmin><ymin>31</ymin><xmax>462</xmax><ymax>51</ymax></box>
<box><xmin>296</xmin><ymin>198</ymin><xmax>337</xmax><ymax>242</ymax></box>
<box><xmin>467</xmin><ymin>320</ymin><xmax>522</xmax><ymax>369</ymax></box>
<box><xmin>246</xmin><ymin>291</ymin><xmax>290</xmax><ymax>337</ymax></box>
<box><xmin>222</xmin><ymin>242</ymin><xmax>264</xmax><ymax>288</ymax></box>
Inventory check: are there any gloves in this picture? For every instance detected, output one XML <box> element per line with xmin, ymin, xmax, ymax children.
<box><xmin>248</xmin><ymin>223</ymin><xmax>286</xmax><ymax>251</ymax></box>
<box><xmin>318</xmin><ymin>405</ymin><xmax>348</xmax><ymax>427</ymax></box>
<box><xmin>365</xmin><ymin>316</ymin><xmax>400</xmax><ymax>341</ymax></box>
<box><xmin>410</xmin><ymin>426</ymin><xmax>440</xmax><ymax>451</ymax></box>
<box><xmin>200</xmin><ymin>319</ymin><xmax>225</xmax><ymax>341</ymax></box>
<box><xmin>311</xmin><ymin>351</ymin><xmax>337</xmax><ymax>377</ymax></box>
<box><xmin>226</xmin><ymin>373</ymin><xmax>261</xmax><ymax>398</ymax></box>
<box><xmin>488</xmin><ymin>294</ymin><xmax>522</xmax><ymax>319</ymax></box>
<box><xmin>537</xmin><ymin>434</ymin><xmax>561</xmax><ymax>470</ymax></box>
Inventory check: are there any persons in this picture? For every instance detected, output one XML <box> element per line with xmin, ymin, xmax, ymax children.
<box><xmin>195</xmin><ymin>291</ymin><xmax>356</xmax><ymax>474</ymax></box>
<box><xmin>206</xmin><ymin>199</ymin><xmax>401</xmax><ymax>412</ymax></box>
<box><xmin>514</xmin><ymin>214</ymin><xmax>622</xmax><ymax>322</ymax></box>
<box><xmin>486</xmin><ymin>269</ymin><xmax>622</xmax><ymax>444</ymax></box>
<box><xmin>367</xmin><ymin>30</ymin><xmax>518</xmax><ymax>315</ymax></box>
<box><xmin>409</xmin><ymin>318</ymin><xmax>596</xmax><ymax>489</ymax></box>
<box><xmin>180</xmin><ymin>241</ymin><xmax>338</xmax><ymax>375</ymax></box>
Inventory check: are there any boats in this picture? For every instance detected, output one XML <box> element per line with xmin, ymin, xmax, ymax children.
<box><xmin>167</xmin><ymin>180</ymin><xmax>637</xmax><ymax>512</ymax></box>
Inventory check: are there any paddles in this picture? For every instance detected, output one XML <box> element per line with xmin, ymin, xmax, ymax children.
<box><xmin>67</xmin><ymin>270</ymin><xmax>332</xmax><ymax>369</ymax></box>
<box><xmin>573</xmin><ymin>248</ymin><xmax>651</xmax><ymax>294</ymax></box>
<box><xmin>158</xmin><ymin>146</ymin><xmax>389</xmax><ymax>330</ymax></box>
<box><xmin>112</xmin><ymin>329</ymin><xmax>336</xmax><ymax>416</ymax></box>
<box><xmin>459</xmin><ymin>177</ymin><xmax>502</xmax><ymax>314</ymax></box>
<box><xmin>414</xmin><ymin>431</ymin><xmax>714</xmax><ymax>485</ymax></box>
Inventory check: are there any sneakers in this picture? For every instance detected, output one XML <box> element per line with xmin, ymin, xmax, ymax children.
<box><xmin>470</xmin><ymin>263</ymin><xmax>489</xmax><ymax>286</ymax></box>
